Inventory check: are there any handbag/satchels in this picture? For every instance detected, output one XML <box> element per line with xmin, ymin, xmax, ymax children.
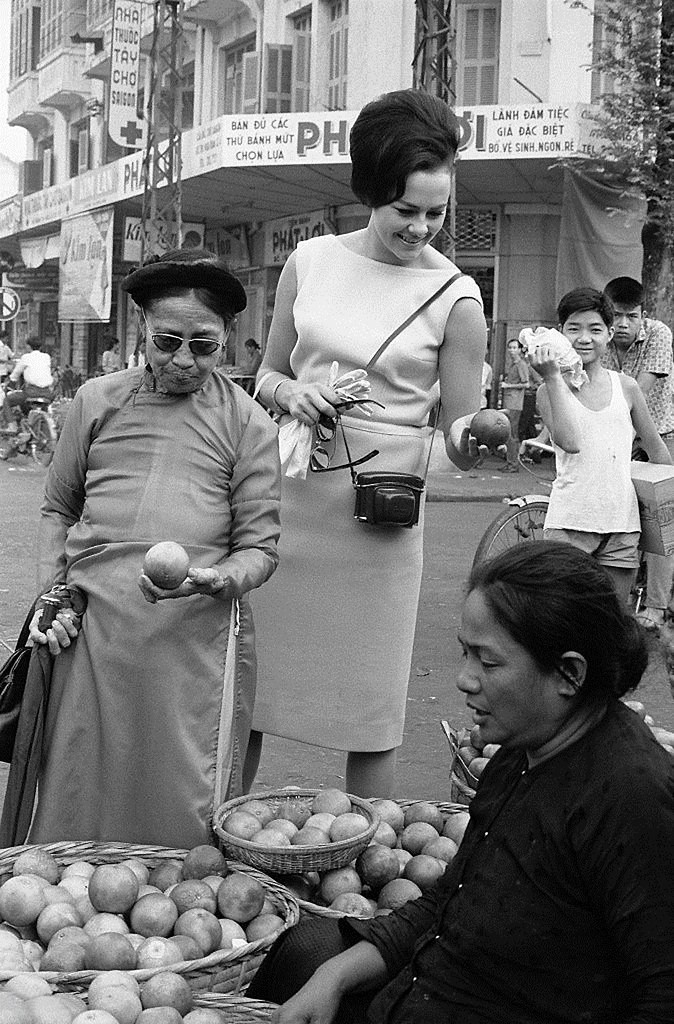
<box><xmin>0</xmin><ymin>585</ymin><xmax>86</xmax><ymax>762</ymax></box>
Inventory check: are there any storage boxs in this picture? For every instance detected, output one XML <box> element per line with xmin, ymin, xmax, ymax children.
<box><xmin>627</xmin><ymin>459</ymin><xmax>674</xmax><ymax>558</ymax></box>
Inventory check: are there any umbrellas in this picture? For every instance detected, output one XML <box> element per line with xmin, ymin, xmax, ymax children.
<box><xmin>0</xmin><ymin>593</ymin><xmax>65</xmax><ymax>849</ymax></box>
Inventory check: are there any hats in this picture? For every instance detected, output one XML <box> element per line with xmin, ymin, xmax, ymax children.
<box><xmin>119</xmin><ymin>248</ymin><xmax>248</xmax><ymax>315</ymax></box>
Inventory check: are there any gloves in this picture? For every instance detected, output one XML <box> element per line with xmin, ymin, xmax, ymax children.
<box><xmin>278</xmin><ymin>362</ymin><xmax>373</xmax><ymax>480</ymax></box>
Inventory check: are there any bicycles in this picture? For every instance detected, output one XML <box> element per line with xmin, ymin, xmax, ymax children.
<box><xmin>469</xmin><ymin>443</ymin><xmax>674</xmax><ymax>632</ymax></box>
<box><xmin>0</xmin><ymin>383</ymin><xmax>59</xmax><ymax>467</ymax></box>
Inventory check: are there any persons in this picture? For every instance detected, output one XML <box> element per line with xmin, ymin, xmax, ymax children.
<box><xmin>26</xmin><ymin>250</ymin><xmax>283</xmax><ymax>849</ymax></box>
<box><xmin>0</xmin><ymin>330</ymin><xmax>55</xmax><ymax>449</ymax></box>
<box><xmin>527</xmin><ymin>277</ymin><xmax>674</xmax><ymax>632</ymax></box>
<box><xmin>242</xmin><ymin>89</ymin><xmax>510</xmax><ymax>798</ymax></box>
<box><xmin>481</xmin><ymin>338</ymin><xmax>543</xmax><ymax>465</ymax></box>
<box><xmin>237</xmin><ymin>338</ymin><xmax>264</xmax><ymax>397</ymax></box>
<box><xmin>245</xmin><ymin>539</ymin><xmax>674</xmax><ymax>1024</ymax></box>
<box><xmin>102</xmin><ymin>336</ymin><xmax>149</xmax><ymax>375</ymax></box>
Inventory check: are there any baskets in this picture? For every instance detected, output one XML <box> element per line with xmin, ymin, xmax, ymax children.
<box><xmin>0</xmin><ymin>718</ymin><xmax>484</xmax><ymax>1024</ymax></box>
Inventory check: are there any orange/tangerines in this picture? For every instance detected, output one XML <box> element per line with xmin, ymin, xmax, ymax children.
<box><xmin>625</xmin><ymin>700</ymin><xmax>674</xmax><ymax>754</ymax></box>
<box><xmin>471</xmin><ymin>408</ymin><xmax>512</xmax><ymax>447</ymax></box>
<box><xmin>0</xmin><ymin>845</ymin><xmax>284</xmax><ymax>1024</ymax></box>
<box><xmin>143</xmin><ymin>541</ymin><xmax>189</xmax><ymax>589</ymax></box>
<box><xmin>223</xmin><ymin>787</ymin><xmax>470</xmax><ymax>916</ymax></box>
<box><xmin>460</xmin><ymin>724</ymin><xmax>502</xmax><ymax>777</ymax></box>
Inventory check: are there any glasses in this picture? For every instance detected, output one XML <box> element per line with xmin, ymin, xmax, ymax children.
<box><xmin>139</xmin><ymin>302</ymin><xmax>232</xmax><ymax>355</ymax></box>
<box><xmin>311</xmin><ymin>399</ymin><xmax>384</xmax><ymax>474</ymax></box>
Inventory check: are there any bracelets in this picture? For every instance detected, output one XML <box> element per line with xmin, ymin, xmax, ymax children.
<box><xmin>272</xmin><ymin>379</ymin><xmax>294</xmax><ymax>413</ymax></box>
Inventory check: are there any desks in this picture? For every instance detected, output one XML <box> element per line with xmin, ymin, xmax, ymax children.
<box><xmin>228</xmin><ymin>376</ymin><xmax>255</xmax><ymax>393</ymax></box>
